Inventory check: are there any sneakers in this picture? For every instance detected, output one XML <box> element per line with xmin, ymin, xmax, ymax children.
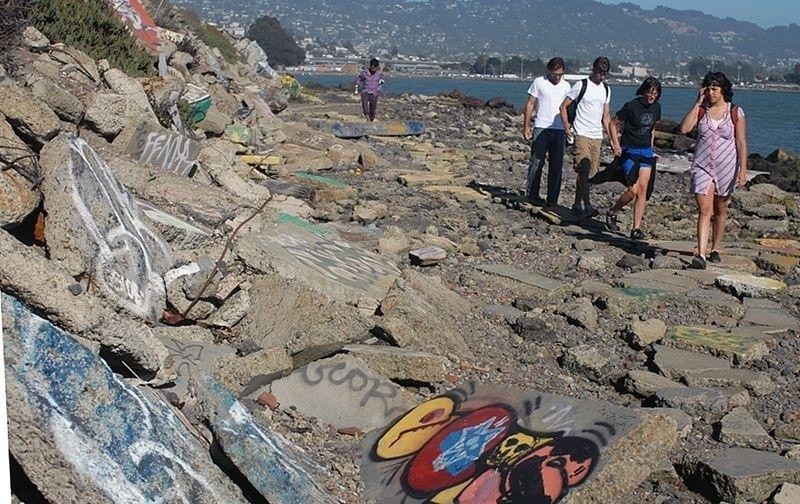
<box><xmin>709</xmin><ymin>250</ymin><xmax>720</xmax><ymax>263</ymax></box>
<box><xmin>527</xmin><ymin>195</ymin><xmax>556</xmax><ymax>208</ymax></box>
<box><xmin>693</xmin><ymin>253</ymin><xmax>706</xmax><ymax>269</ymax></box>
<box><xmin>571</xmin><ymin>204</ymin><xmax>600</xmax><ymax>218</ymax></box>
<box><xmin>606</xmin><ymin>209</ymin><xmax>619</xmax><ymax>232</ymax></box>
<box><xmin>630</xmin><ymin>228</ymin><xmax>647</xmax><ymax>240</ymax></box>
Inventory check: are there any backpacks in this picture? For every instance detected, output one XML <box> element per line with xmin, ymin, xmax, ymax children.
<box><xmin>567</xmin><ymin>79</ymin><xmax>608</xmax><ymax>123</ymax></box>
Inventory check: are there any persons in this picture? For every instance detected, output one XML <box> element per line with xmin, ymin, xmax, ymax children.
<box><xmin>354</xmin><ymin>59</ymin><xmax>385</xmax><ymax>121</ymax></box>
<box><xmin>679</xmin><ymin>71</ymin><xmax>747</xmax><ymax>269</ymax></box>
<box><xmin>605</xmin><ymin>77</ymin><xmax>662</xmax><ymax>240</ymax></box>
<box><xmin>559</xmin><ymin>57</ymin><xmax>616</xmax><ymax>219</ymax></box>
<box><xmin>524</xmin><ymin>57</ymin><xmax>571</xmax><ymax>207</ymax></box>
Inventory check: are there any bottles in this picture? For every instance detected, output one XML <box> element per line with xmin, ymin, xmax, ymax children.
<box><xmin>567</xmin><ymin>125</ymin><xmax>576</xmax><ymax>145</ymax></box>
<box><xmin>354</xmin><ymin>83</ymin><xmax>359</xmax><ymax>96</ymax></box>
